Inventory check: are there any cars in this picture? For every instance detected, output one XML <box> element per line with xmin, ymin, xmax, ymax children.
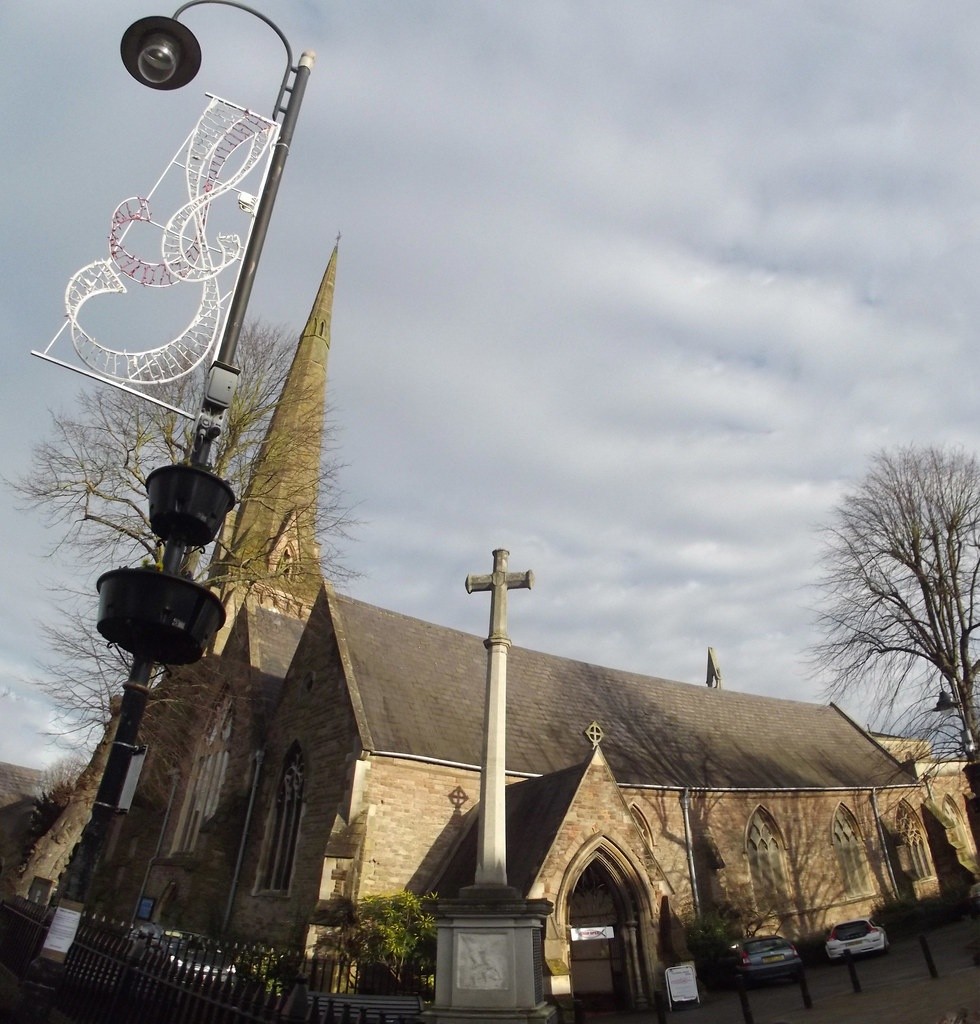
<box><xmin>825</xmin><ymin>918</ymin><xmax>889</xmax><ymax>961</ymax></box>
<box><xmin>125</xmin><ymin>922</ymin><xmax>239</xmax><ymax>999</ymax></box>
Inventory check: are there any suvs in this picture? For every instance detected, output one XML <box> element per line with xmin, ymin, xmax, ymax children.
<box><xmin>699</xmin><ymin>934</ymin><xmax>804</xmax><ymax>992</ymax></box>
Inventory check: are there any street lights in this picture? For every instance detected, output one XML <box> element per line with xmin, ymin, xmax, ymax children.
<box><xmin>927</xmin><ymin>672</ymin><xmax>974</xmax><ymax>764</ymax></box>
<box><xmin>5</xmin><ymin>0</ymin><xmax>318</xmax><ymax>1024</ymax></box>
